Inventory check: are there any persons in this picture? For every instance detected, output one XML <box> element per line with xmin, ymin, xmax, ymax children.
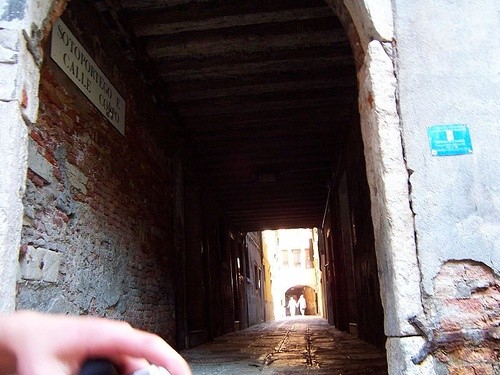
<box><xmin>0</xmin><ymin>308</ymin><xmax>192</xmax><ymax>375</ymax></box>
<box><xmin>297</xmin><ymin>294</ymin><xmax>307</xmax><ymax>316</ymax></box>
<box><xmin>287</xmin><ymin>296</ymin><xmax>297</xmax><ymax>316</ymax></box>
